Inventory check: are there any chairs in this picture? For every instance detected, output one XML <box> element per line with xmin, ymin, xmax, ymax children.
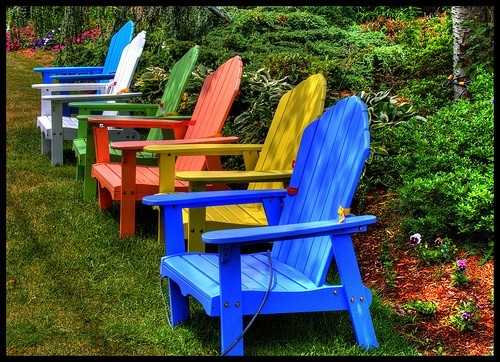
<box><xmin>33</xmin><ymin>20</ymin><xmax>135</xmax><ymax>117</ymax></box>
<box><xmin>30</xmin><ymin>28</ymin><xmax>143</xmax><ymax>166</ymax></box>
<box><xmin>67</xmin><ymin>44</ymin><xmax>201</xmax><ymax>203</ymax></box>
<box><xmin>142</xmin><ymin>72</ymin><xmax>327</xmax><ymax>262</ymax></box>
<box><xmin>140</xmin><ymin>94</ymin><xmax>382</xmax><ymax>356</ymax></box>
<box><xmin>86</xmin><ymin>55</ymin><xmax>244</xmax><ymax>240</ymax></box>
